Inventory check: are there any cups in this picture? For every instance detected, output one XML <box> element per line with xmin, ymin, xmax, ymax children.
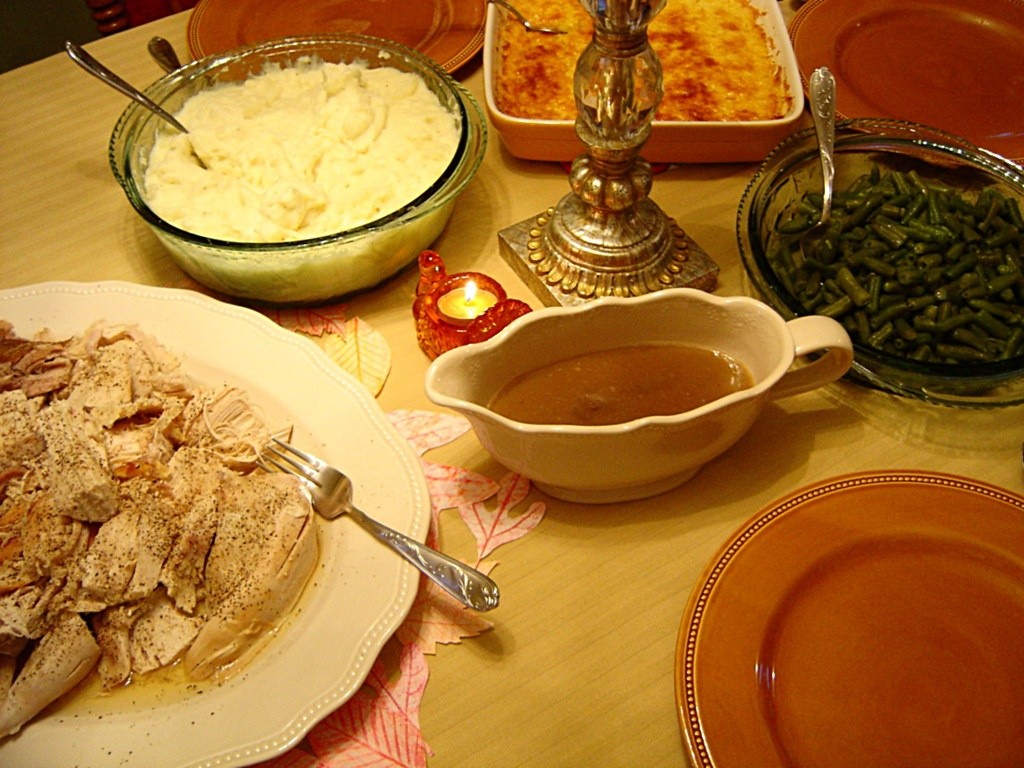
<box><xmin>424</xmin><ymin>287</ymin><xmax>855</xmax><ymax>506</ymax></box>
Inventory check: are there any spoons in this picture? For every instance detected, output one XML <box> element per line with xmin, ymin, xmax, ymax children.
<box><xmin>486</xmin><ymin>0</ymin><xmax>568</xmax><ymax>35</ymax></box>
<box><xmin>63</xmin><ymin>40</ymin><xmax>208</xmax><ymax>174</ymax></box>
<box><xmin>797</xmin><ymin>65</ymin><xmax>844</xmax><ymax>270</ymax></box>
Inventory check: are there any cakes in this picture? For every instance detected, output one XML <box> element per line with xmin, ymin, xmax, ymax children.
<box><xmin>493</xmin><ymin>0</ymin><xmax>793</xmax><ymax>121</ymax></box>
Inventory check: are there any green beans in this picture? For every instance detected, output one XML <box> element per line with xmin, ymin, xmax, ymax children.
<box><xmin>769</xmin><ymin>165</ymin><xmax>1024</xmax><ymax>395</ymax></box>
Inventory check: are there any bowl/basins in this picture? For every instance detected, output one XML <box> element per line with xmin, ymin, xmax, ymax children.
<box><xmin>107</xmin><ymin>34</ymin><xmax>486</xmax><ymax>308</ymax></box>
<box><xmin>734</xmin><ymin>117</ymin><xmax>1024</xmax><ymax>406</ymax></box>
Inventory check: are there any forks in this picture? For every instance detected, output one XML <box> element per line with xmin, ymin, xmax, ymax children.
<box><xmin>254</xmin><ymin>437</ymin><xmax>501</xmax><ymax>614</ymax></box>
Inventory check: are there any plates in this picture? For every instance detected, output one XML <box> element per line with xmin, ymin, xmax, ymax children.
<box><xmin>184</xmin><ymin>0</ymin><xmax>489</xmax><ymax>86</ymax></box>
<box><xmin>673</xmin><ymin>469</ymin><xmax>1024</xmax><ymax>768</ymax></box>
<box><xmin>785</xmin><ymin>0</ymin><xmax>1024</xmax><ymax>165</ymax></box>
<box><xmin>482</xmin><ymin>0</ymin><xmax>805</xmax><ymax>164</ymax></box>
<box><xmin>0</xmin><ymin>280</ymin><xmax>432</xmax><ymax>768</ymax></box>
